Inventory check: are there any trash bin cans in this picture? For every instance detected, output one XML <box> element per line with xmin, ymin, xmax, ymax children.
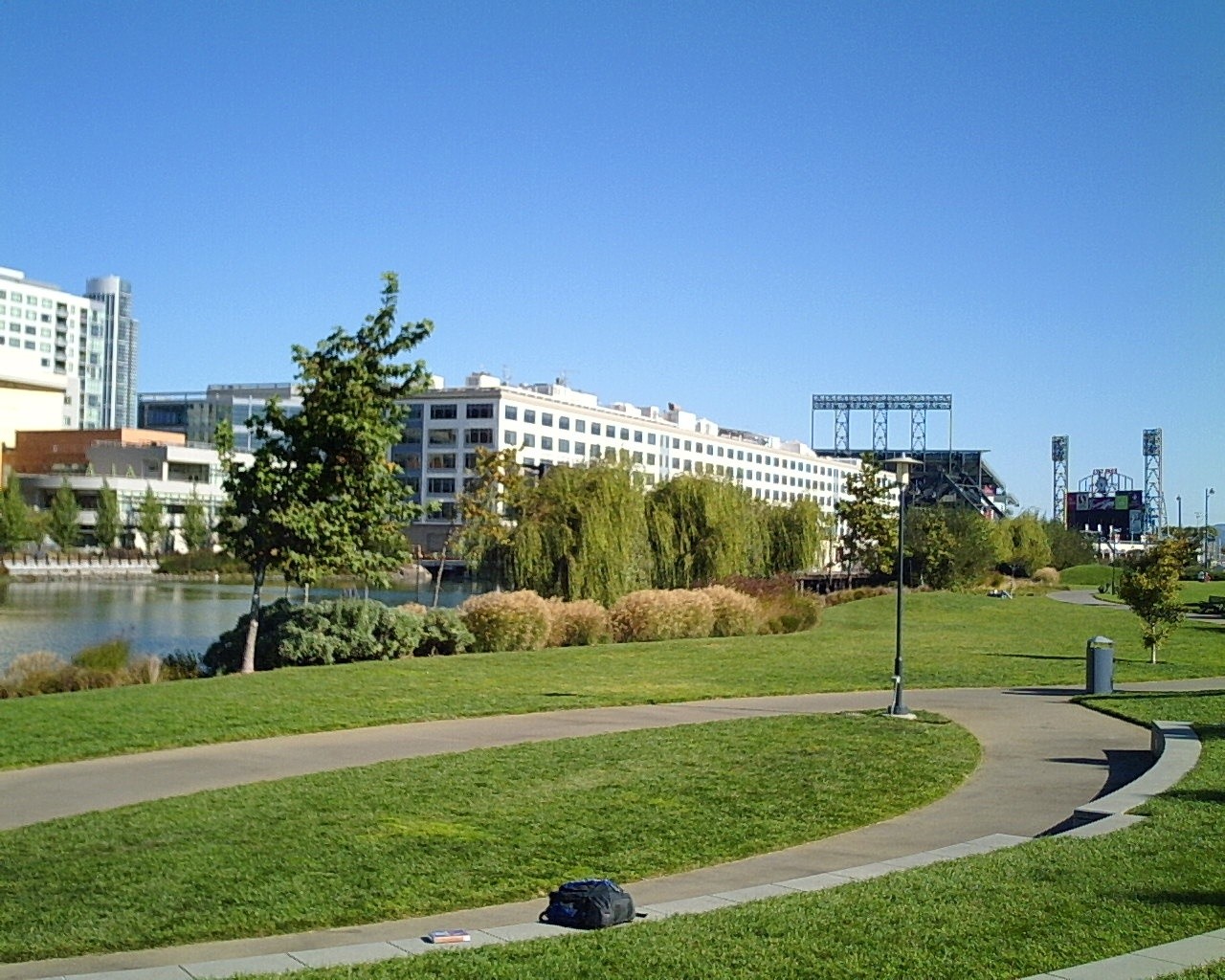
<box><xmin>1087</xmin><ymin>635</ymin><xmax>1115</xmax><ymax>693</ymax></box>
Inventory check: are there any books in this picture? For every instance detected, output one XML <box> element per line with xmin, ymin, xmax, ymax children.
<box><xmin>427</xmin><ymin>929</ymin><xmax>471</xmax><ymax>944</ymax></box>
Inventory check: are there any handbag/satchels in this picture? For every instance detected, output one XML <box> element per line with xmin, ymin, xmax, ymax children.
<box><xmin>539</xmin><ymin>879</ymin><xmax>635</xmax><ymax>929</ymax></box>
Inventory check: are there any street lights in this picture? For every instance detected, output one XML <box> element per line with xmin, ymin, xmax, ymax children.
<box><xmin>1176</xmin><ymin>494</ymin><xmax>1183</xmax><ymax>529</ymax></box>
<box><xmin>881</xmin><ymin>451</ymin><xmax>924</xmax><ymax>711</ymax></box>
<box><xmin>1204</xmin><ymin>488</ymin><xmax>1215</xmax><ymax>569</ymax></box>
